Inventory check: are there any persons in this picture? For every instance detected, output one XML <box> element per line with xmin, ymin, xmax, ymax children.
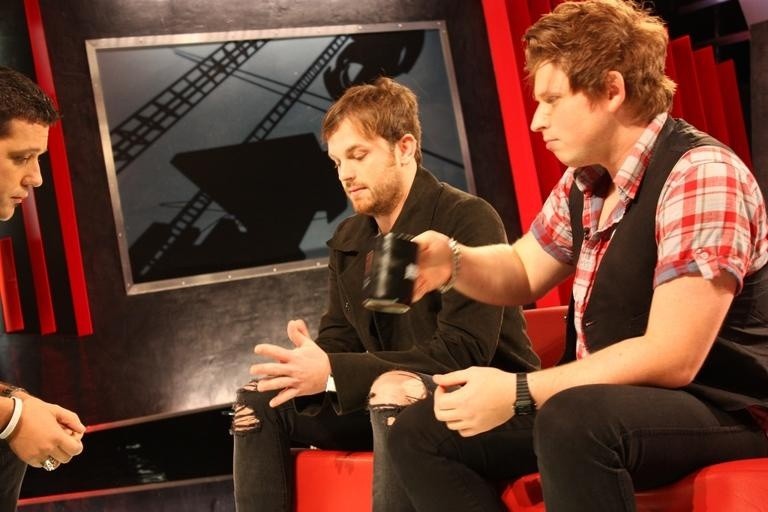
<box><xmin>0</xmin><ymin>63</ymin><xmax>87</xmax><ymax>511</ymax></box>
<box><xmin>407</xmin><ymin>0</ymin><xmax>768</xmax><ymax>512</ymax></box>
<box><xmin>223</xmin><ymin>77</ymin><xmax>541</xmax><ymax>511</ymax></box>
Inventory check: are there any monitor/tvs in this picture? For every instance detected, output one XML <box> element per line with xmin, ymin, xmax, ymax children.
<box><xmin>84</xmin><ymin>18</ymin><xmax>479</xmax><ymax>301</ymax></box>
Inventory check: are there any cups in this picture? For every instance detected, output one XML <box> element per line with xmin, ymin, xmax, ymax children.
<box><xmin>359</xmin><ymin>232</ymin><xmax>416</xmax><ymax>316</ymax></box>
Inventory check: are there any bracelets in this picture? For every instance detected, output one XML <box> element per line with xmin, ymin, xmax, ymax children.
<box><xmin>0</xmin><ymin>397</ymin><xmax>23</xmax><ymax>440</ymax></box>
<box><xmin>1</xmin><ymin>383</ymin><xmax>25</xmax><ymax>397</ymax></box>
<box><xmin>326</xmin><ymin>373</ymin><xmax>338</xmax><ymax>393</ymax></box>
<box><xmin>439</xmin><ymin>235</ymin><xmax>462</xmax><ymax>295</ymax></box>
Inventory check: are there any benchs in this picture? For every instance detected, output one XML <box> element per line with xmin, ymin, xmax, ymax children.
<box><xmin>288</xmin><ymin>303</ymin><xmax>766</xmax><ymax>511</ymax></box>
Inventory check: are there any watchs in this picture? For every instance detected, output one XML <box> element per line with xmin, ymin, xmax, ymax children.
<box><xmin>513</xmin><ymin>371</ymin><xmax>536</xmax><ymax>417</ymax></box>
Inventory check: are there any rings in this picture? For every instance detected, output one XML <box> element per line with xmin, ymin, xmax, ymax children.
<box><xmin>40</xmin><ymin>454</ymin><xmax>58</xmax><ymax>472</ymax></box>
